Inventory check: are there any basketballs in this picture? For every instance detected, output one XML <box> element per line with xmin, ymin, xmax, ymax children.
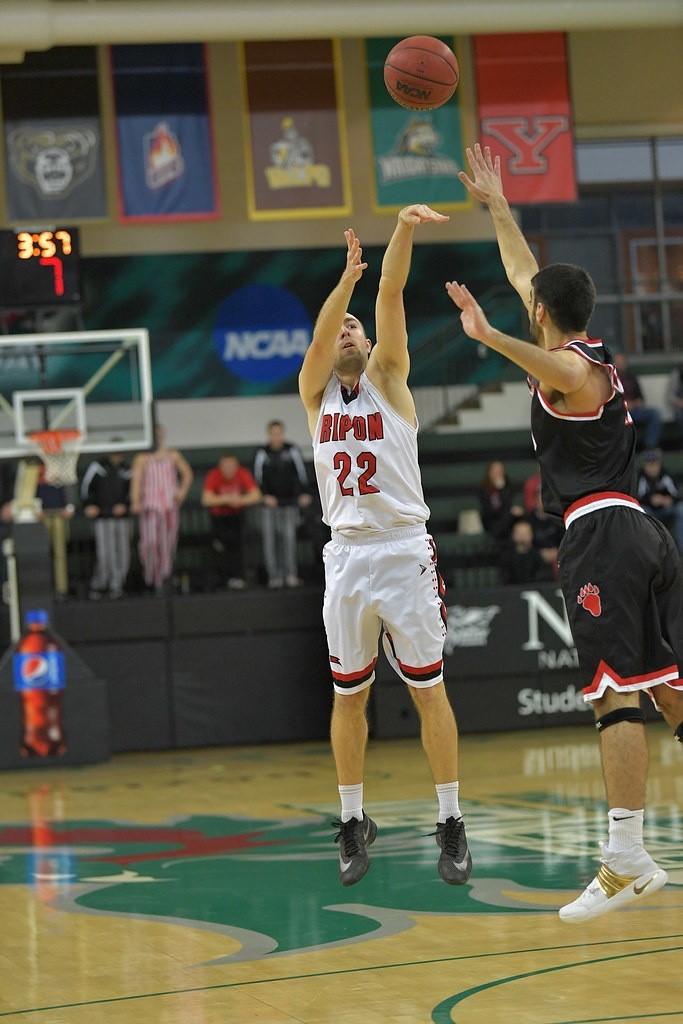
<box><xmin>382</xmin><ymin>33</ymin><xmax>461</xmax><ymax>113</ymax></box>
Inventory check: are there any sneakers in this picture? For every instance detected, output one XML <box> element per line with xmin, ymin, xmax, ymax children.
<box><xmin>559</xmin><ymin>840</ymin><xmax>669</xmax><ymax>924</ymax></box>
<box><xmin>435</xmin><ymin>817</ymin><xmax>473</xmax><ymax>885</ymax></box>
<box><xmin>331</xmin><ymin>809</ymin><xmax>378</xmax><ymax>887</ymax></box>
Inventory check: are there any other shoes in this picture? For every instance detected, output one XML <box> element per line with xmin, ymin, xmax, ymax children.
<box><xmin>225</xmin><ymin>580</ymin><xmax>248</xmax><ymax>592</ymax></box>
<box><xmin>90</xmin><ymin>589</ymin><xmax>123</xmax><ymax>601</ymax></box>
<box><xmin>270</xmin><ymin>576</ymin><xmax>300</xmax><ymax>589</ymax></box>
<box><xmin>147</xmin><ymin>586</ymin><xmax>163</xmax><ymax>594</ymax></box>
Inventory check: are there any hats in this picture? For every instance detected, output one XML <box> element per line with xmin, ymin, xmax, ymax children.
<box><xmin>642</xmin><ymin>447</ymin><xmax>664</xmax><ymax>461</ymax></box>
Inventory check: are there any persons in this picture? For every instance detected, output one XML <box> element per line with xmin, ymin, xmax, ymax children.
<box><xmin>445</xmin><ymin>142</ymin><xmax>683</xmax><ymax>925</ymax></box>
<box><xmin>613</xmin><ymin>350</ymin><xmax>662</xmax><ymax>448</ymax></box>
<box><xmin>298</xmin><ymin>204</ymin><xmax>473</xmax><ymax>886</ymax></box>
<box><xmin>484</xmin><ymin>454</ymin><xmax>543</xmax><ymax>536</ymax></box>
<box><xmin>129</xmin><ymin>423</ymin><xmax>193</xmax><ymax>599</ymax></box>
<box><xmin>636</xmin><ymin>448</ymin><xmax>678</xmax><ymax>511</ymax></box>
<box><xmin>254</xmin><ymin>421</ymin><xmax>308</xmax><ymax>589</ymax></box>
<box><xmin>666</xmin><ymin>359</ymin><xmax>683</xmax><ymax>426</ymax></box>
<box><xmin>201</xmin><ymin>453</ymin><xmax>263</xmax><ymax>593</ymax></box>
<box><xmin>81</xmin><ymin>437</ymin><xmax>134</xmax><ymax>602</ymax></box>
<box><xmin>498</xmin><ymin>520</ymin><xmax>555</xmax><ymax>585</ymax></box>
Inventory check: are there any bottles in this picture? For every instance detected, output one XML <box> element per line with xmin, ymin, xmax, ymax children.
<box><xmin>14</xmin><ymin>607</ymin><xmax>69</xmax><ymax>758</ymax></box>
